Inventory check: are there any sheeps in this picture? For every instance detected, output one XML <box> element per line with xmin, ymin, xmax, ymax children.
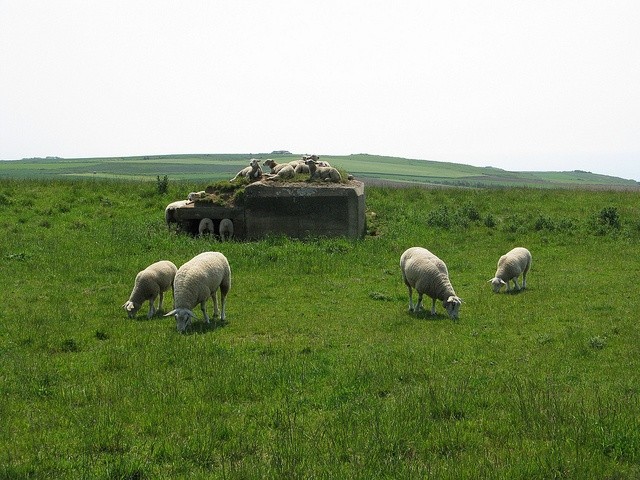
<box><xmin>186</xmin><ymin>191</ymin><xmax>206</xmax><ymax>205</ymax></box>
<box><xmin>230</xmin><ymin>158</ymin><xmax>262</xmax><ymax>182</ymax></box>
<box><xmin>163</xmin><ymin>251</ymin><xmax>231</xmax><ymax>334</ymax></box>
<box><xmin>398</xmin><ymin>246</ymin><xmax>466</xmax><ymax>320</ymax></box>
<box><xmin>219</xmin><ymin>219</ymin><xmax>233</xmax><ymax>242</ymax></box>
<box><xmin>304</xmin><ymin>159</ymin><xmax>342</xmax><ymax>183</ymax></box>
<box><xmin>262</xmin><ymin>159</ymin><xmax>295</xmax><ymax>181</ymax></box>
<box><xmin>199</xmin><ymin>218</ymin><xmax>214</xmax><ymax>236</ymax></box>
<box><xmin>121</xmin><ymin>260</ymin><xmax>177</xmax><ymax>319</ymax></box>
<box><xmin>487</xmin><ymin>247</ymin><xmax>532</xmax><ymax>294</ymax></box>
<box><xmin>294</xmin><ymin>160</ymin><xmax>330</xmax><ymax>173</ymax></box>
<box><xmin>289</xmin><ymin>154</ymin><xmax>320</xmax><ymax>169</ymax></box>
